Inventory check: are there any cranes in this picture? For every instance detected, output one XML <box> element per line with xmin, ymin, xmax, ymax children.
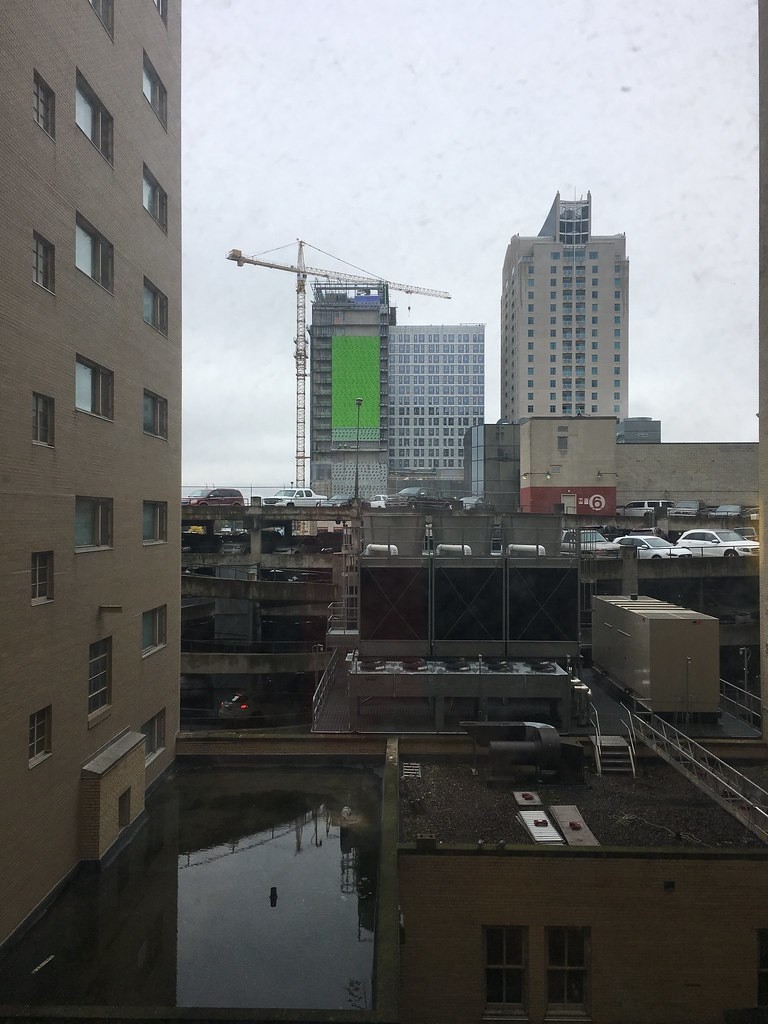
<box><xmin>226</xmin><ymin>238</ymin><xmax>451</xmax><ymax>490</ymax></box>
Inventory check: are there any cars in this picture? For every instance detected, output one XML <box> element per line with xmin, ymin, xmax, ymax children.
<box><xmin>181</xmin><ymin>488</ymin><xmax>245</xmax><ymax>506</ymax></box>
<box><xmin>562</xmin><ymin>525</ymin><xmax>760</xmax><ymax>559</ymax></box>
<box><xmin>181</xmin><ymin>529</ymin><xmax>321</xmax><ymax>555</ymax></box>
<box><xmin>214</xmin><ymin>689</ymin><xmax>286</xmax><ymax>728</ymax></box>
<box><xmin>616</xmin><ymin>499</ymin><xmax>759</xmax><ymax>520</ymax></box>
<box><xmin>365</xmin><ymin>486</ymin><xmax>486</xmax><ymax>510</ymax></box>
<box><xmin>262</xmin><ymin>488</ymin><xmax>353</xmax><ymax>507</ymax></box>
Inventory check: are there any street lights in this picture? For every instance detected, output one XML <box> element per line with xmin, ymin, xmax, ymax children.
<box><xmin>739</xmin><ymin>647</ymin><xmax>751</xmax><ymax>722</ymax></box>
<box><xmin>354</xmin><ymin>397</ymin><xmax>363</xmax><ymax>499</ymax></box>
